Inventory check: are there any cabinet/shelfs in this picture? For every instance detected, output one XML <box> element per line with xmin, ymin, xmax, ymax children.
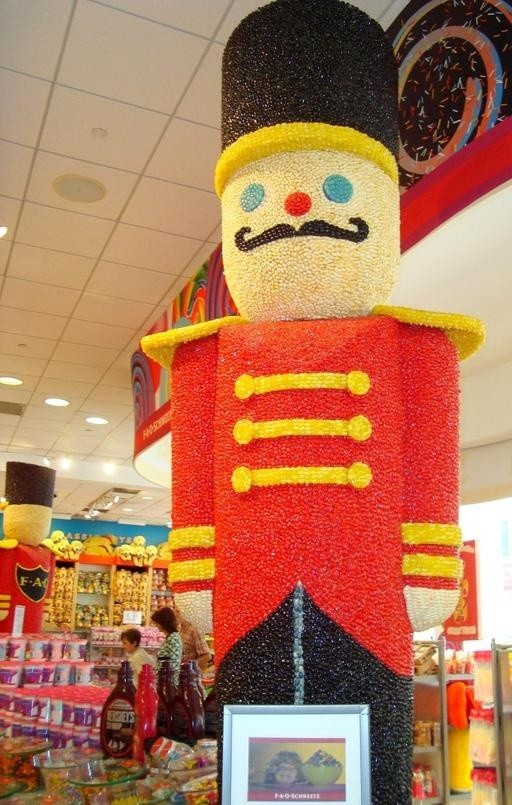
<box><xmin>44</xmin><ymin>552</ymin><xmax>173</xmax><ymax>631</ymax></box>
<box><xmin>90</xmin><ymin>635</ymin><xmax>164</xmax><ymax>686</ymax></box>
<box><xmin>408</xmin><ymin>637</ymin><xmax>474</xmax><ymax>804</ymax></box>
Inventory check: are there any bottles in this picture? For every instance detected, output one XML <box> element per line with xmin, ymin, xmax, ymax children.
<box><xmin>136</xmin><ymin>665</ymin><xmax>158</xmax><ymax>762</ymax></box>
<box><xmin>158</xmin><ymin>662</ymin><xmax>175</xmax><ymax>738</ymax></box>
<box><xmin>204</xmin><ymin>686</ymin><xmax>220</xmax><ymax>739</ymax></box>
<box><xmin>101</xmin><ymin>661</ymin><xmax>136</xmax><ymax>758</ymax></box>
<box><xmin>190</xmin><ymin>661</ymin><xmax>205</xmax><ymax>704</ymax></box>
<box><xmin>174</xmin><ymin>664</ymin><xmax>204</xmax><ymax>747</ymax></box>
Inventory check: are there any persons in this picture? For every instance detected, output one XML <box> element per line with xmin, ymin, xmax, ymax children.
<box><xmin>122</xmin><ymin>629</ymin><xmax>153</xmax><ymax>690</ymax></box>
<box><xmin>151</xmin><ymin>607</ymin><xmax>181</xmax><ymax>691</ymax></box>
<box><xmin>174</xmin><ymin>608</ymin><xmax>212</xmax><ymax>671</ymax></box>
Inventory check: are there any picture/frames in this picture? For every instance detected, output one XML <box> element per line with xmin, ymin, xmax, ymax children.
<box><xmin>219</xmin><ymin>703</ymin><xmax>372</xmax><ymax>805</ymax></box>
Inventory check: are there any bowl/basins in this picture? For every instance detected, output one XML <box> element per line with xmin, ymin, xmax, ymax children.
<box><xmin>299</xmin><ymin>765</ymin><xmax>342</xmax><ymax>789</ymax></box>
